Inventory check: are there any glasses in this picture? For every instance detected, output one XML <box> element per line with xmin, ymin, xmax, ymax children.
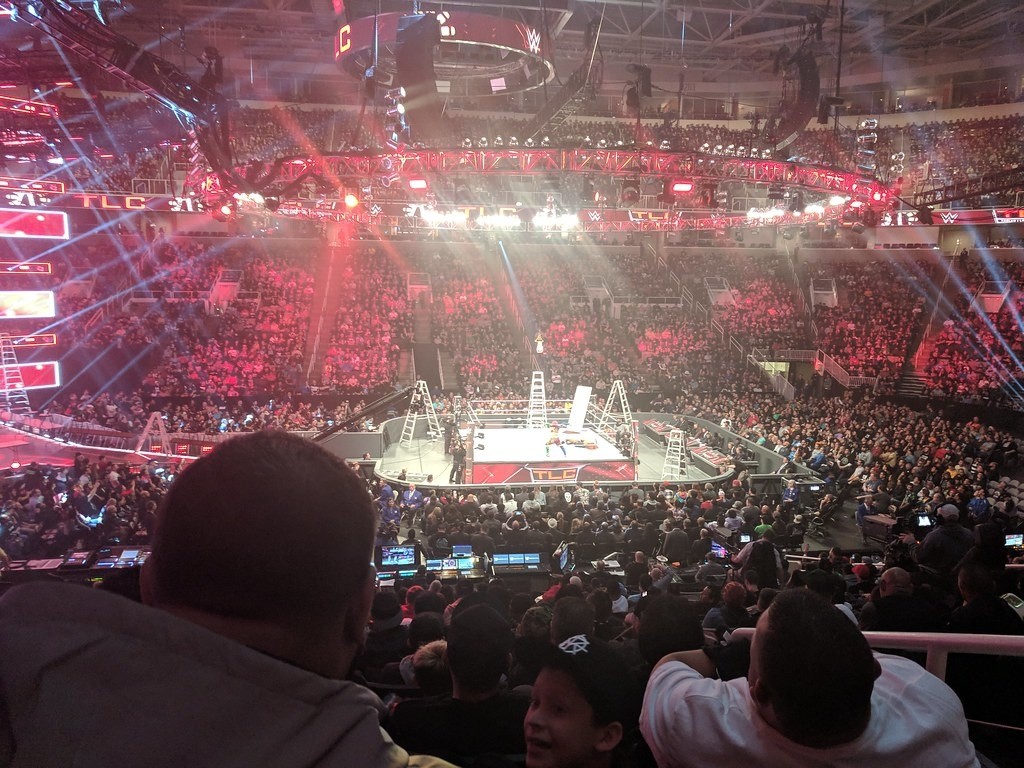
<box><xmin>359</xmin><ymin>564</ymin><xmax>381</xmax><ymax>593</ymax></box>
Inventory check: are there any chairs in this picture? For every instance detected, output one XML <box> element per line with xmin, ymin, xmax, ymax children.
<box><xmin>687</xmin><ymin>420</ymin><xmax>755</xmax><ymax>462</ymax></box>
<box><xmin>919</xmin><ymin>522</ymin><xmax>1006</xmax><ymax>600</ymax></box>
<box><xmin>851</xmin><ymin>495</ymin><xmax>873</xmax><ymax>544</ymax></box>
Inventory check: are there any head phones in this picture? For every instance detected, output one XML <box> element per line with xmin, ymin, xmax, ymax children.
<box><xmin>933</xmin><ymin>501</ymin><xmax>957</xmax><ymax>525</ymax></box>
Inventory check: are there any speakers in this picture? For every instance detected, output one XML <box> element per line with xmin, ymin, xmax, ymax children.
<box><xmin>394</xmin><ymin>15</ymin><xmax>455</xmax><ymax>144</ymax></box>
<box><xmin>762</xmin><ymin>49</ymin><xmax>821</xmax><ymax>155</ymax></box>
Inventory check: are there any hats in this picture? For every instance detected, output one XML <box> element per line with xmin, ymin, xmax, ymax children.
<box><xmin>852</xmin><ymin>565</ymin><xmax>870</xmax><ymax>580</ymax></box>
<box><xmin>937</xmin><ymin>504</ymin><xmax>960</xmax><ymax>519</ymax></box>
<box><xmin>732</xmin><ymin>500</ymin><xmax>743</xmax><ymax>507</ymax></box>
<box><xmin>398</xmin><ymin>653</ymin><xmax>416</xmax><ymax>689</ymax></box>
<box><xmin>430</xmin><ymin>579</ymin><xmax>442</xmax><ymax>589</ymax></box>
<box><xmin>987</xmin><ymin>497</ymin><xmax>996</xmax><ymax>506</ymax></box>
<box><xmin>511</xmin><ymin>632</ymin><xmax>659</xmax><ymax>724</ymax></box>
<box><xmin>732</xmin><ymin>480</ymin><xmax>742</xmax><ymax>487</ymax></box>
<box><xmin>547</xmin><ymin>518</ymin><xmax>558</xmax><ymax>528</ymax></box>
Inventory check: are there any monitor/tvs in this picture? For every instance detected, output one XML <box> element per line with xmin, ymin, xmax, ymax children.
<box><xmin>381</xmin><ymin>545</ymin><xmax>416</xmax><ymax>565</ymax></box>
<box><xmin>493</xmin><ymin>553</ymin><xmax>541</xmax><ymax>566</ymax></box>
<box><xmin>427</xmin><ymin>546</ymin><xmax>476</xmax><ymax>574</ymax></box>
<box><xmin>558</xmin><ymin>543</ymin><xmax>569</xmax><ymax>571</ymax></box>
<box><xmin>120</xmin><ymin>550</ymin><xmax>139</xmax><ymax>559</ymax></box>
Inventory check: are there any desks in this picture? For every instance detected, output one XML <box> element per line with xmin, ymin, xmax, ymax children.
<box><xmin>863</xmin><ymin>515</ymin><xmax>898</xmax><ymax>550</ymax></box>
<box><xmin>642</xmin><ymin>423</ymin><xmax>728</xmax><ymax>476</ymax></box>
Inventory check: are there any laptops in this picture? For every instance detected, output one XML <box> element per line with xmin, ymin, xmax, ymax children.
<box><xmin>1004</xmin><ymin>534</ymin><xmax>1023</xmax><ymax>546</ymax></box>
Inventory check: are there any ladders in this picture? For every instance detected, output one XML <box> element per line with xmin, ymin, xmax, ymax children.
<box><xmin>526</xmin><ymin>371</ymin><xmax>547</xmax><ymax>429</ymax></box>
<box><xmin>0</xmin><ymin>332</ymin><xmax>34</xmax><ymax>427</ymax></box>
<box><xmin>466</xmin><ymin>402</ymin><xmax>482</xmax><ymax>427</ymax></box>
<box><xmin>398</xmin><ymin>381</ymin><xmax>442</xmax><ymax>449</ymax></box>
<box><xmin>135</xmin><ymin>412</ymin><xmax>173</xmax><ymax>459</ymax></box>
<box><xmin>379</xmin><ymin>467</ymin><xmax>432</xmax><ymax>481</ymax></box>
<box><xmin>598</xmin><ymin>380</ymin><xmax>635</xmax><ymax>440</ymax></box>
<box><xmin>661</xmin><ymin>429</ymin><xmax>688</xmax><ymax>481</ymax></box>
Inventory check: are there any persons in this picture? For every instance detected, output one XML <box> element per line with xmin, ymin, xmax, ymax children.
<box><xmin>0</xmin><ymin>72</ymin><xmax>1024</xmax><ymax>768</ymax></box>
<box><xmin>0</xmin><ymin>428</ymin><xmax>460</xmax><ymax>768</ymax></box>
<box><xmin>514</xmin><ymin>634</ymin><xmax>633</xmax><ymax>768</ymax></box>
<box><xmin>638</xmin><ymin>589</ymin><xmax>982</xmax><ymax>768</ymax></box>
